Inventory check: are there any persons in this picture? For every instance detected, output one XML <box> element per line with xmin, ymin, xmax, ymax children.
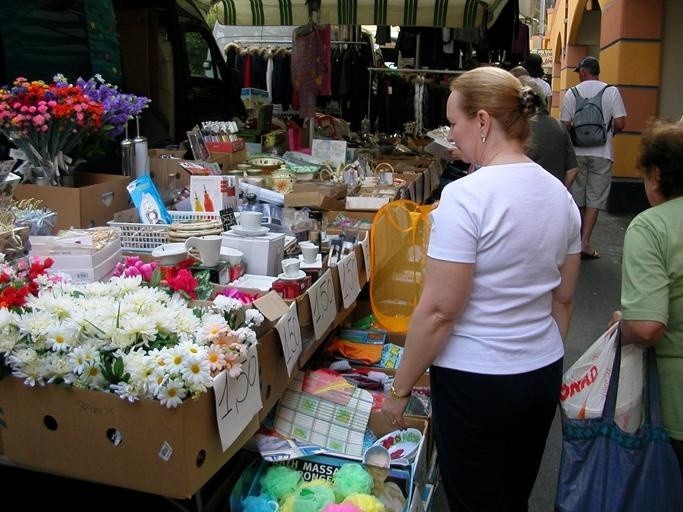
<box><xmin>518</xmin><ymin>76</ymin><xmax>578</xmax><ymax>190</ymax></box>
<box><xmin>559</xmin><ymin>56</ymin><xmax>627</xmax><ymax>260</ymax></box>
<box><xmin>381</xmin><ymin>65</ymin><xmax>582</xmax><ymax>512</ymax></box>
<box><xmin>608</xmin><ymin>115</ymin><xmax>682</xmax><ymax>511</ymax></box>
<box><xmin>377</xmin><ymin>58</ymin><xmax>388</xmax><ymax>68</ymax></box>
<box><xmin>521</xmin><ymin>53</ymin><xmax>553</xmax><ymax>115</ymax></box>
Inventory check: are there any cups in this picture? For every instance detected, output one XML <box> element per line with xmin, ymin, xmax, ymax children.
<box><xmin>184</xmin><ymin>211</ymin><xmax>319</xmax><ymax>278</ymax></box>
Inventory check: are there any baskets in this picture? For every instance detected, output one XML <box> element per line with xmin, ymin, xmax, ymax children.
<box><xmin>106</xmin><ymin>210</ymin><xmax>240</xmax><ymax>253</ymax></box>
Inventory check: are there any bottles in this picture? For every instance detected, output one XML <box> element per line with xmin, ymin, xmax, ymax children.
<box><xmin>227</xmin><ymin>163</ymin><xmax>297</xmax><ymax>194</ymax></box>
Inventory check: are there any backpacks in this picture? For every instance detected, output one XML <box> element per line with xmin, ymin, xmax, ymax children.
<box><xmin>569</xmin><ymin>84</ymin><xmax>616</xmax><ymax>148</ymax></box>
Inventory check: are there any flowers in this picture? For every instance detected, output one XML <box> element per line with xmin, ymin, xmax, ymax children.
<box><xmin>0</xmin><ymin>78</ymin><xmax>105</xmax><ymax>177</ymax></box>
<box><xmin>53</xmin><ymin>73</ymin><xmax>152</xmax><ymax>176</ymax></box>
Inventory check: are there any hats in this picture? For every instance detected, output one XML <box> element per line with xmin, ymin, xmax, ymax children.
<box><xmin>572</xmin><ymin>56</ymin><xmax>600</xmax><ymax>74</ymax></box>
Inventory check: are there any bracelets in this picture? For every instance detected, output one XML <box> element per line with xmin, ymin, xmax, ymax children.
<box><xmin>386</xmin><ymin>377</ymin><xmax>412</xmax><ymax>400</ymax></box>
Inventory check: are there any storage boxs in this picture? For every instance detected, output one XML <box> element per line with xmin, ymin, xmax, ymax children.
<box><xmin>179</xmin><ymin>132</ymin><xmax>252</xmax><ymax>173</ymax></box>
<box><xmin>145</xmin><ymin>149</ymin><xmax>230</xmax><ymax>196</ymax></box>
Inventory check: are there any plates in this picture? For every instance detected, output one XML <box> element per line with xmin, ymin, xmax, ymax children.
<box><xmin>277</xmin><ymin>270</ymin><xmax>306</xmax><ymax>281</ymax></box>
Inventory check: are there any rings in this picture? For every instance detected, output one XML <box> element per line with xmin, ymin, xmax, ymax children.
<box><xmin>390</xmin><ymin>418</ymin><xmax>396</xmax><ymax>424</ymax></box>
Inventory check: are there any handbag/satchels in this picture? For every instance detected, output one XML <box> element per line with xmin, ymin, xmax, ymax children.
<box><xmin>553</xmin><ymin>416</ymin><xmax>683</xmax><ymax>512</ymax></box>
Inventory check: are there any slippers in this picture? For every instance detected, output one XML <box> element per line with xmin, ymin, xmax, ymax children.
<box><xmin>580</xmin><ymin>250</ymin><xmax>601</xmax><ymax>260</ymax></box>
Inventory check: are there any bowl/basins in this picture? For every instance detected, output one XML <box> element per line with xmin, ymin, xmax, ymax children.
<box><xmin>362</xmin><ymin>427</ymin><xmax>423</xmax><ymax>465</ymax></box>
<box><xmin>151</xmin><ymin>242</ymin><xmax>192</xmax><ymax>266</ymax></box>
